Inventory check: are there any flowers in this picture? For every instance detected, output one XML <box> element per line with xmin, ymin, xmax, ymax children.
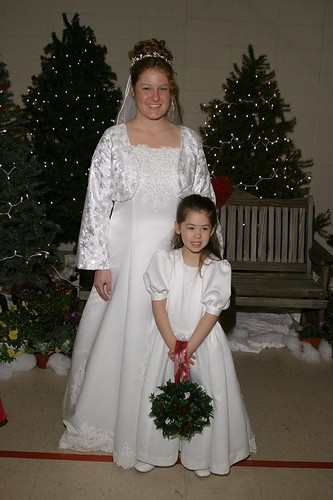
<box><xmin>148</xmin><ymin>379</ymin><xmax>215</xmax><ymax>443</ymax></box>
<box><xmin>0</xmin><ymin>289</ymin><xmax>77</xmax><ymax>364</ymax></box>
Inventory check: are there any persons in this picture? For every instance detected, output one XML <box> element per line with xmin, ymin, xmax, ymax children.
<box><xmin>131</xmin><ymin>193</ymin><xmax>234</xmax><ymax>477</ymax></box>
<box><xmin>58</xmin><ymin>39</ymin><xmax>259</xmax><ymax>455</ymax></box>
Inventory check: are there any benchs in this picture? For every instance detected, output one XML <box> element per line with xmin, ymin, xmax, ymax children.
<box><xmin>79</xmin><ymin>196</ymin><xmax>328</xmax><ymax>331</ymax></box>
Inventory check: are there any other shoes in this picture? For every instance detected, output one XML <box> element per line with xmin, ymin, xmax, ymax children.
<box><xmin>195</xmin><ymin>469</ymin><xmax>210</xmax><ymax>477</ymax></box>
<box><xmin>135</xmin><ymin>462</ymin><xmax>154</xmax><ymax>472</ymax></box>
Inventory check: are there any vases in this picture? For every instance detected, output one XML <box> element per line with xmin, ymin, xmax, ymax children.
<box><xmin>35</xmin><ymin>351</ymin><xmax>53</xmax><ymax>369</ymax></box>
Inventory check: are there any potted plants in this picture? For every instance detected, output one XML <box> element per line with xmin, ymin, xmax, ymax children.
<box><xmin>295</xmin><ymin>322</ymin><xmax>321</xmax><ymax>353</ymax></box>
